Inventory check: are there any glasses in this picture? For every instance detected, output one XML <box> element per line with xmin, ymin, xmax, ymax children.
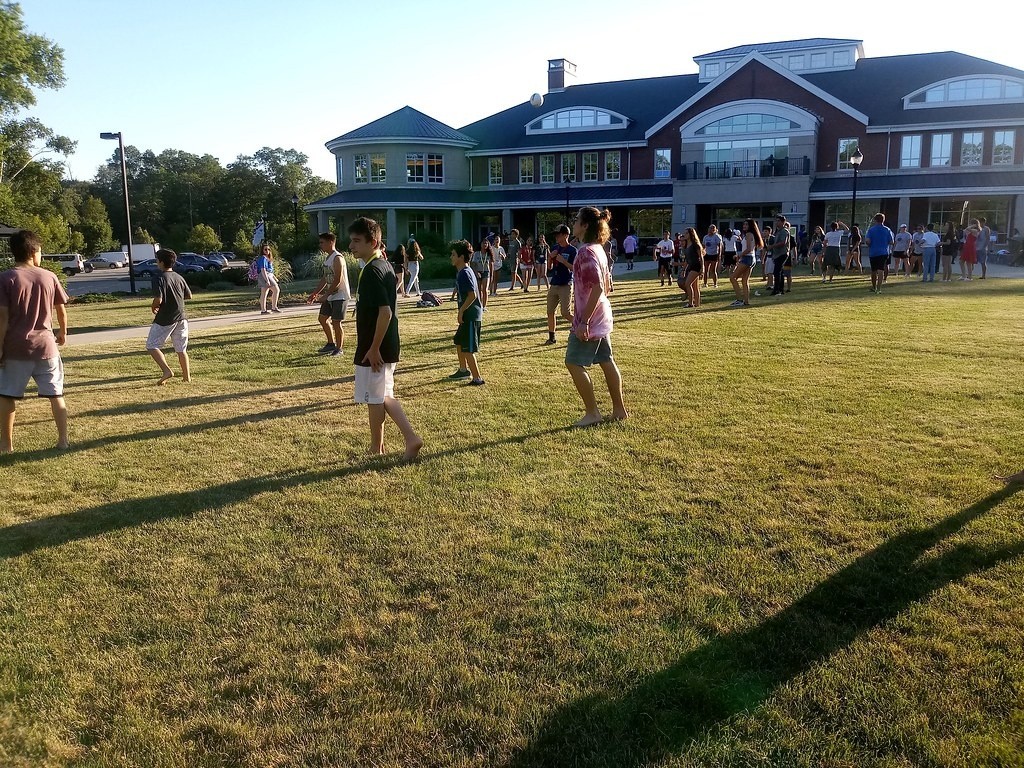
<box><xmin>264</xmin><ymin>249</ymin><xmax>270</xmax><ymax>251</ymax></box>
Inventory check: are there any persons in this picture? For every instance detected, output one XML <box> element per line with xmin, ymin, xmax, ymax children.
<box><xmin>654</xmin><ymin>213</ymin><xmax>990</xmax><ymax>307</ymax></box>
<box><xmin>256</xmin><ymin>244</ymin><xmax>283</xmax><ymax>314</ymax></box>
<box><xmin>448</xmin><ymin>239</ymin><xmax>485</xmax><ymax>386</ymax></box>
<box><xmin>347</xmin><ymin>217</ymin><xmax>423</xmax><ymax>463</ymax></box>
<box><xmin>390</xmin><ymin>243</ymin><xmax>406</xmax><ymax>297</ymax></box>
<box><xmin>307</xmin><ymin>232</ymin><xmax>350</xmax><ymax>356</ymax></box>
<box><xmin>146</xmin><ymin>249</ymin><xmax>192</xmax><ymax>384</ymax></box>
<box><xmin>604</xmin><ymin>228</ymin><xmax>637</xmax><ymax>292</ymax></box>
<box><xmin>0</xmin><ymin>230</ymin><xmax>69</xmax><ymax>450</ymax></box>
<box><xmin>472</xmin><ymin>224</ymin><xmax>580</xmax><ymax>345</ymax></box>
<box><xmin>564</xmin><ymin>206</ymin><xmax>629</xmax><ymax>426</ymax></box>
<box><xmin>358</xmin><ymin>241</ymin><xmax>386</xmax><ymax>270</ymax></box>
<box><xmin>404</xmin><ymin>234</ymin><xmax>424</xmax><ymax>298</ymax></box>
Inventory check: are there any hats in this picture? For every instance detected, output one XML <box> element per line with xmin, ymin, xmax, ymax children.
<box><xmin>900</xmin><ymin>224</ymin><xmax>906</xmax><ymax>228</ymax></box>
<box><xmin>550</xmin><ymin>224</ymin><xmax>571</xmax><ymax>234</ymax></box>
<box><xmin>489</xmin><ymin>232</ymin><xmax>495</xmax><ymax>236</ymax></box>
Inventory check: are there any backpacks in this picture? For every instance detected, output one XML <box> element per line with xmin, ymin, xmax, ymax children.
<box><xmin>417</xmin><ymin>300</ymin><xmax>434</xmax><ymax>308</ymax></box>
<box><xmin>421</xmin><ymin>291</ymin><xmax>443</xmax><ymax>306</ymax></box>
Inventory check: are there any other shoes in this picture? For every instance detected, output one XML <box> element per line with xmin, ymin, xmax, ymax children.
<box><xmin>682</xmin><ymin>303</ymin><xmax>692</xmax><ymax>308</ymax></box>
<box><xmin>543</xmin><ymin>340</ymin><xmax>556</xmax><ymax>346</ymax></box>
<box><xmin>978</xmin><ymin>277</ymin><xmax>986</xmax><ymax>280</ymax></box>
<box><xmin>261</xmin><ymin>311</ymin><xmax>270</xmax><ymax>315</ymax></box>
<box><xmin>964</xmin><ymin>277</ymin><xmax>973</xmax><ymax>281</ymax></box>
<box><xmin>956</xmin><ymin>276</ymin><xmax>965</xmax><ymax>280</ymax></box>
<box><xmin>271</xmin><ymin>307</ymin><xmax>283</xmax><ymax>314</ymax></box>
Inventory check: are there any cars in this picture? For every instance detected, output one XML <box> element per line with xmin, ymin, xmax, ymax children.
<box><xmin>85</xmin><ymin>257</ymin><xmax>123</xmax><ymax>269</ymax></box>
<box><xmin>128</xmin><ymin>257</ymin><xmax>206</xmax><ymax>278</ymax></box>
<box><xmin>83</xmin><ymin>261</ymin><xmax>96</xmax><ymax>273</ymax></box>
<box><xmin>177</xmin><ymin>251</ymin><xmax>237</xmax><ymax>273</ymax></box>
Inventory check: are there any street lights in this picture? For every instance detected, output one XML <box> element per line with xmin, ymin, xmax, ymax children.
<box><xmin>291</xmin><ymin>191</ymin><xmax>299</xmax><ymax>255</ymax></box>
<box><xmin>100</xmin><ymin>132</ymin><xmax>136</xmax><ymax>293</ymax></box>
<box><xmin>849</xmin><ymin>145</ymin><xmax>864</xmax><ymax>247</ymax></box>
<box><xmin>563</xmin><ymin>175</ymin><xmax>572</xmax><ymax>227</ymax></box>
<box><xmin>261</xmin><ymin>211</ymin><xmax>268</xmax><ymax>244</ymax></box>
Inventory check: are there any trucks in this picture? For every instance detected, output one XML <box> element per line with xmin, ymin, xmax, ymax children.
<box><xmin>91</xmin><ymin>251</ymin><xmax>129</xmax><ymax>267</ymax></box>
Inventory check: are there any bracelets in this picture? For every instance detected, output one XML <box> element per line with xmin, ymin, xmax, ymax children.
<box><xmin>580</xmin><ymin>321</ymin><xmax>588</xmax><ymax>325</ymax></box>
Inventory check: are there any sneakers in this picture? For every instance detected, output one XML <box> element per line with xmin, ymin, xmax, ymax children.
<box><xmin>459</xmin><ymin>380</ymin><xmax>485</xmax><ymax>387</ymax></box>
<box><xmin>318</xmin><ymin>343</ymin><xmax>336</xmax><ymax>354</ymax></box>
<box><xmin>730</xmin><ymin>300</ymin><xmax>745</xmax><ymax>306</ymax></box>
<box><xmin>330</xmin><ymin>349</ymin><xmax>343</xmax><ymax>358</ymax></box>
<box><xmin>449</xmin><ymin>369</ymin><xmax>470</xmax><ymax>380</ymax></box>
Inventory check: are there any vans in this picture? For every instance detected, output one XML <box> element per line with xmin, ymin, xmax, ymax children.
<box><xmin>41</xmin><ymin>254</ymin><xmax>85</xmax><ymax>276</ymax></box>
